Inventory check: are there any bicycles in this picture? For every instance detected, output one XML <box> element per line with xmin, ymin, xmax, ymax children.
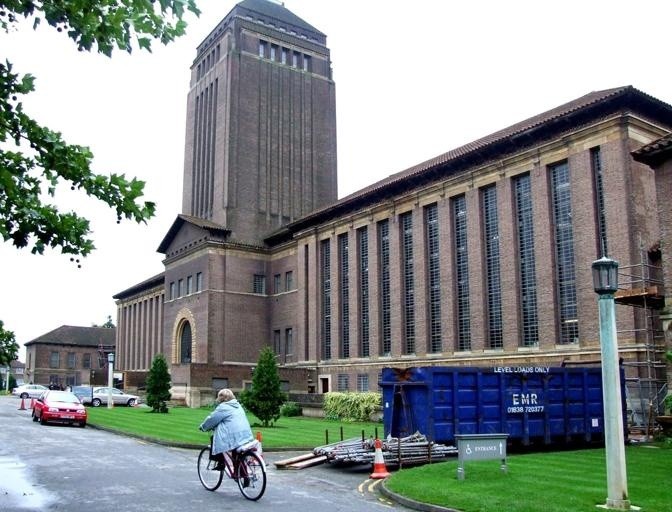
<box><xmin>198</xmin><ymin>428</ymin><xmax>266</xmax><ymax>501</ymax></box>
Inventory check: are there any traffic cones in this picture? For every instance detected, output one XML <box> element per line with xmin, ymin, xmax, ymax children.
<box><xmin>247</xmin><ymin>432</ymin><xmax>264</xmax><ymax>465</ymax></box>
<box><xmin>369</xmin><ymin>438</ymin><xmax>391</xmax><ymax>478</ymax></box>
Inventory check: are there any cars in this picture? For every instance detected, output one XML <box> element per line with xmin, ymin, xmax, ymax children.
<box><xmin>12</xmin><ymin>384</ymin><xmax>141</xmax><ymax>427</ymax></box>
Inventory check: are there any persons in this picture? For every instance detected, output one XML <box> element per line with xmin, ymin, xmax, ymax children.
<box><xmin>199</xmin><ymin>388</ymin><xmax>254</xmax><ymax>488</ymax></box>
<box><xmin>49</xmin><ymin>381</ymin><xmax>71</xmax><ymax>392</ymax></box>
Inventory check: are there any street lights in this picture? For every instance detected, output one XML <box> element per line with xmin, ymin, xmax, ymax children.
<box><xmin>108</xmin><ymin>353</ymin><xmax>114</xmax><ymax>408</ymax></box>
<box><xmin>592</xmin><ymin>237</ymin><xmax>642</xmax><ymax>511</ymax></box>
<box><xmin>6</xmin><ymin>366</ymin><xmax>10</xmax><ymax>393</ymax></box>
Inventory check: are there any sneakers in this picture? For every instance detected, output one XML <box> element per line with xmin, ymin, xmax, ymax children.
<box><xmin>211</xmin><ymin>463</ymin><xmax>225</xmax><ymax>471</ymax></box>
